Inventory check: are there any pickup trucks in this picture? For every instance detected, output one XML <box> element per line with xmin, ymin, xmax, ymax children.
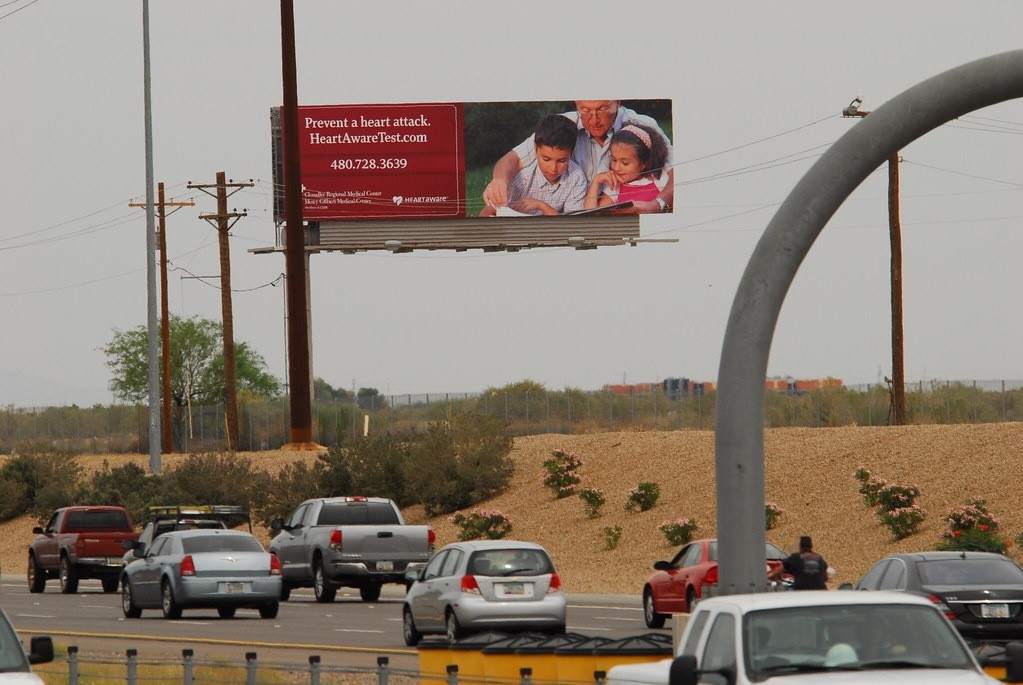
<box><xmin>267</xmin><ymin>495</ymin><xmax>435</xmax><ymax>604</ymax></box>
<box><xmin>27</xmin><ymin>505</ymin><xmax>143</xmax><ymax>594</ymax></box>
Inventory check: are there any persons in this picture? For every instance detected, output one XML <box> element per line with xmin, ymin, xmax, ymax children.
<box><xmin>584</xmin><ymin>124</ymin><xmax>669</xmax><ymax>210</ymax></box>
<box><xmin>483</xmin><ymin>100</ymin><xmax>673</xmax><ymax>214</ymax></box>
<box><xmin>766</xmin><ymin>536</ymin><xmax>835</xmax><ymax>590</ymax></box>
<box><xmin>479</xmin><ymin>115</ymin><xmax>587</xmax><ymax>216</ymax></box>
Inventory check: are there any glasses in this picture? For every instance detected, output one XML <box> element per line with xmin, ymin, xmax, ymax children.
<box><xmin>577</xmin><ymin>100</ymin><xmax>615</xmax><ymax>120</ymax></box>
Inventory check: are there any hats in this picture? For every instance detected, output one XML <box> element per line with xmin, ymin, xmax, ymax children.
<box><xmin>800</xmin><ymin>537</ymin><xmax>811</xmax><ymax>546</ymax></box>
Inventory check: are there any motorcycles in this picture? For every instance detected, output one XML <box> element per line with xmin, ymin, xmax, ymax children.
<box><xmin>766</xmin><ymin>562</ymin><xmax>853</xmax><ymax>591</ymax></box>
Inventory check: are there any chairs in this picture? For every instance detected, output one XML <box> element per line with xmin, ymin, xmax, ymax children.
<box><xmin>508</xmin><ymin>558</ymin><xmax>537</xmax><ymax>574</ymax></box>
<box><xmin>476</xmin><ymin>558</ymin><xmax>491</xmax><ymax>572</ymax></box>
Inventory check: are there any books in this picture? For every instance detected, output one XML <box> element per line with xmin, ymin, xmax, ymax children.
<box><xmin>497</xmin><ymin>200</ymin><xmax>633</xmax><ymax>216</ymax></box>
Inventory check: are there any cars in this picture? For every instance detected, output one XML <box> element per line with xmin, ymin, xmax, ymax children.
<box><xmin>839</xmin><ymin>551</ymin><xmax>1023</xmax><ymax>682</ymax></box>
<box><xmin>641</xmin><ymin>538</ymin><xmax>796</xmax><ymax>629</ymax></box>
<box><xmin>0</xmin><ymin>609</ymin><xmax>56</xmax><ymax>685</ymax></box>
<box><xmin>401</xmin><ymin>540</ymin><xmax>567</xmax><ymax>647</ymax></box>
<box><xmin>605</xmin><ymin>591</ymin><xmax>1007</xmax><ymax>685</ymax></box>
<box><xmin>120</xmin><ymin>529</ymin><xmax>283</xmax><ymax>620</ymax></box>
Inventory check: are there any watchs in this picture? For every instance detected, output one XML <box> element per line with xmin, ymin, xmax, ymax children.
<box><xmin>656</xmin><ymin>198</ymin><xmax>669</xmax><ymax>213</ymax></box>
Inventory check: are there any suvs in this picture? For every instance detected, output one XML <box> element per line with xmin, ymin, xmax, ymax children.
<box><xmin>121</xmin><ymin>507</ymin><xmax>229</xmax><ymax>575</ymax></box>
